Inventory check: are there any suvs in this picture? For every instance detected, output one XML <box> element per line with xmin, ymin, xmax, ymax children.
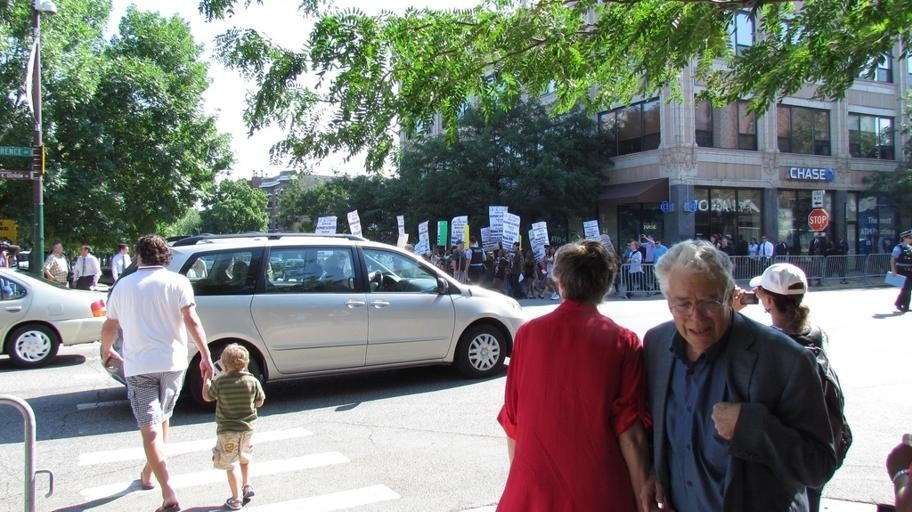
<box><xmin>103</xmin><ymin>230</ymin><xmax>531</xmax><ymax>410</ymax></box>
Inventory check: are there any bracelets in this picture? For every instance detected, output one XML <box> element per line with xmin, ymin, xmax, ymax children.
<box><xmin>892</xmin><ymin>470</ymin><xmax>910</xmax><ymax>484</ymax></box>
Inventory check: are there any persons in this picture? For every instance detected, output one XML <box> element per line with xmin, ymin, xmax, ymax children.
<box><xmin>0</xmin><ymin>241</ymin><xmax>11</xmax><ymax>287</ymax></box>
<box><xmin>43</xmin><ymin>243</ymin><xmax>68</xmax><ymax>287</ymax></box>
<box><xmin>202</xmin><ymin>344</ymin><xmax>265</xmax><ymax>510</ymax></box>
<box><xmin>890</xmin><ymin>229</ymin><xmax>912</xmax><ymax>311</ymax></box>
<box><xmin>112</xmin><ymin>243</ymin><xmax>131</xmax><ymax>281</ymax></box>
<box><xmin>886</xmin><ymin>434</ymin><xmax>911</xmax><ymax>511</ymax></box>
<box><xmin>99</xmin><ymin>234</ymin><xmax>215</xmax><ymax>512</ymax></box>
<box><xmin>496</xmin><ymin>237</ymin><xmax>653</xmax><ymax>511</ymax></box>
<box><xmin>729</xmin><ymin>262</ymin><xmax>853</xmax><ymax>511</ymax></box>
<box><xmin>72</xmin><ymin>245</ymin><xmax>102</xmax><ymax>291</ymax></box>
<box><xmin>639</xmin><ymin>237</ymin><xmax>837</xmax><ymax>510</ymax></box>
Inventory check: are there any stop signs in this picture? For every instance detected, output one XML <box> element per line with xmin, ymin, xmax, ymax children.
<box><xmin>807</xmin><ymin>208</ymin><xmax>830</xmax><ymax>234</ymax></box>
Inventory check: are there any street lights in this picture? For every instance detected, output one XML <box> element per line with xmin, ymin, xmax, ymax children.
<box><xmin>29</xmin><ymin>0</ymin><xmax>60</xmax><ymax>280</ymax></box>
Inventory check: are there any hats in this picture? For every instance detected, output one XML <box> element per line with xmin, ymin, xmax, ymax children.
<box><xmin>455</xmin><ymin>238</ymin><xmax>464</xmax><ymax>244</ymax></box>
<box><xmin>749</xmin><ymin>262</ymin><xmax>811</xmax><ymax>296</ymax></box>
<box><xmin>900</xmin><ymin>229</ymin><xmax>912</xmax><ymax>238</ymax></box>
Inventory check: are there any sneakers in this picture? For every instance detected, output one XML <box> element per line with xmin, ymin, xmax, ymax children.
<box><xmin>529</xmin><ymin>293</ymin><xmax>561</xmax><ymax>301</ymax></box>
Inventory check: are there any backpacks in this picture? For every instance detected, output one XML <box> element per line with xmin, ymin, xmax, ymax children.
<box><xmin>789</xmin><ymin>324</ymin><xmax>853</xmax><ymax>487</ymax></box>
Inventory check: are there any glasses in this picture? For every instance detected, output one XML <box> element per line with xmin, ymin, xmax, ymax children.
<box><xmin>669</xmin><ymin>278</ymin><xmax>731</xmax><ymax>316</ymax></box>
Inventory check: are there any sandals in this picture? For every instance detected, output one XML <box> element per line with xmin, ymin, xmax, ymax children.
<box><xmin>140</xmin><ymin>471</ymin><xmax>155</xmax><ymax>491</ymax></box>
<box><xmin>155</xmin><ymin>502</ymin><xmax>181</xmax><ymax>512</ymax></box>
<box><xmin>225</xmin><ymin>497</ymin><xmax>243</xmax><ymax>511</ymax></box>
<box><xmin>243</xmin><ymin>484</ymin><xmax>255</xmax><ymax>499</ymax></box>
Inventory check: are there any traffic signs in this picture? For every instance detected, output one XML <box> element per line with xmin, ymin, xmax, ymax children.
<box><xmin>0</xmin><ymin>146</ymin><xmax>34</xmax><ymax>157</ymax></box>
<box><xmin>811</xmin><ymin>190</ymin><xmax>826</xmax><ymax>209</ymax></box>
<box><xmin>0</xmin><ymin>170</ymin><xmax>32</xmax><ymax>180</ymax></box>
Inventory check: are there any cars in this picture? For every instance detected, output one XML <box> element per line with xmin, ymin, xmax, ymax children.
<box><xmin>0</xmin><ymin>264</ymin><xmax>110</xmax><ymax>369</ymax></box>
<box><xmin>16</xmin><ymin>250</ymin><xmax>30</xmax><ymax>271</ymax></box>
<box><xmin>197</xmin><ymin>253</ymin><xmax>305</xmax><ymax>283</ymax></box>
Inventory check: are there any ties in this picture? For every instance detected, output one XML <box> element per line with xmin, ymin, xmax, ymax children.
<box><xmin>121</xmin><ymin>255</ymin><xmax>125</xmax><ymax>272</ymax></box>
<box><xmin>81</xmin><ymin>258</ymin><xmax>85</xmax><ymax>275</ymax></box>
